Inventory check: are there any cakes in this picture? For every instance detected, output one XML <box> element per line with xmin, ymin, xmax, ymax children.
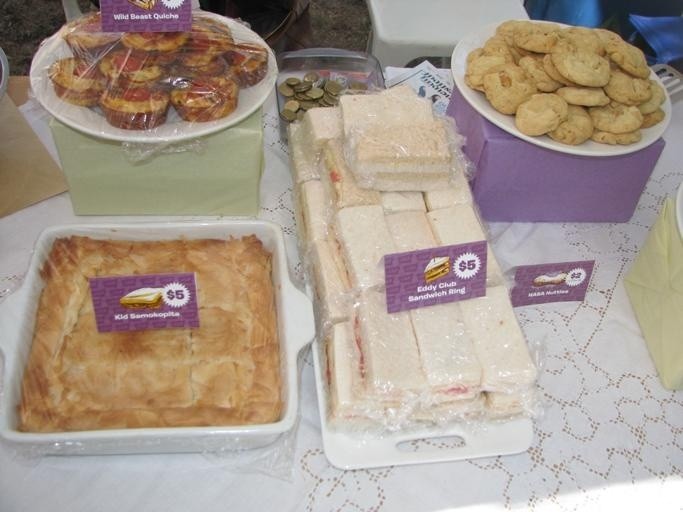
<box><xmin>47</xmin><ymin>13</ymin><xmax>269</xmax><ymax>131</ymax></box>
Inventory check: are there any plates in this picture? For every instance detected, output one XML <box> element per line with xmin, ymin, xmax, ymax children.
<box><xmin>1</xmin><ymin>224</ymin><xmax>315</xmax><ymax>458</ymax></box>
<box><xmin>30</xmin><ymin>10</ymin><xmax>279</xmax><ymax>143</ymax></box>
<box><xmin>304</xmin><ymin>283</ymin><xmax>534</xmax><ymax>471</ymax></box>
<box><xmin>452</xmin><ymin>20</ymin><xmax>673</xmax><ymax>158</ymax></box>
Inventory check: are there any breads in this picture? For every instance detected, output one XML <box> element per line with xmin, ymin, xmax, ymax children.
<box><xmin>18</xmin><ymin>235</ymin><xmax>281</xmax><ymax>432</ymax></box>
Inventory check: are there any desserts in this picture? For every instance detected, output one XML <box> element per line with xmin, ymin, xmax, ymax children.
<box><xmin>287</xmin><ymin>86</ymin><xmax>538</xmax><ymax>432</ymax></box>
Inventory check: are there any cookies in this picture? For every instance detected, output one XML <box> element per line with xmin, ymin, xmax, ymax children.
<box><xmin>279</xmin><ymin>71</ymin><xmax>368</xmax><ymax>121</ymax></box>
<box><xmin>465</xmin><ymin>20</ymin><xmax>665</xmax><ymax>145</ymax></box>
